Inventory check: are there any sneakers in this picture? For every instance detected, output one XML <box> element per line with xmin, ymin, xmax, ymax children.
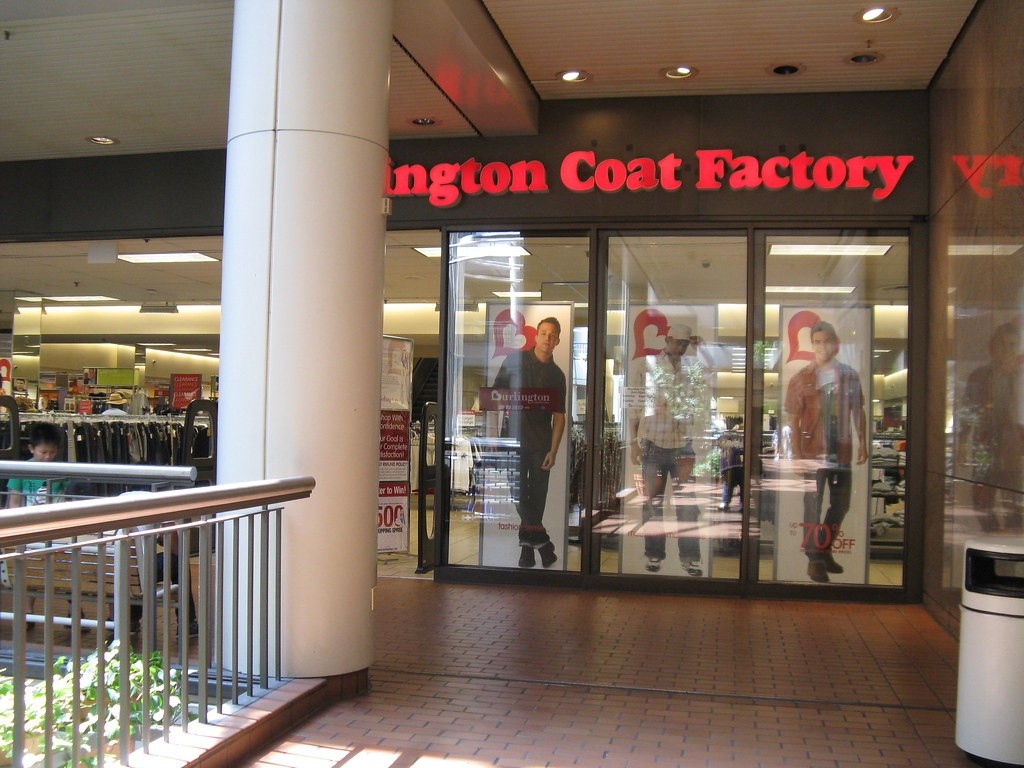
<box><xmin>680</xmin><ymin>558</ymin><xmax>704</xmax><ymax>576</ymax></box>
<box><xmin>646</xmin><ymin>556</ymin><xmax>662</xmax><ymax>572</ymax></box>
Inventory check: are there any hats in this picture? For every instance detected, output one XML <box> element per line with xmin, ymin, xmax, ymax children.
<box><xmin>667</xmin><ymin>324</ymin><xmax>694</xmax><ymax>342</ymax></box>
<box><xmin>106</xmin><ymin>394</ymin><xmax>127</xmax><ymax>405</ymax></box>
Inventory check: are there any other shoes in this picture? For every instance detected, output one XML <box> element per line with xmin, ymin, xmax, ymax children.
<box><xmin>808</xmin><ymin>556</ymin><xmax>843</xmax><ymax>583</ymax></box>
<box><xmin>519</xmin><ymin>545</ymin><xmax>536</xmax><ymax>567</ymax></box>
<box><xmin>538</xmin><ymin>541</ymin><xmax>557</xmax><ymax>568</ymax></box>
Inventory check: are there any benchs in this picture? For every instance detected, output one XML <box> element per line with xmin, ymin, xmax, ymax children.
<box><xmin>0</xmin><ymin>547</ymin><xmax>180</xmax><ymax>632</ymax></box>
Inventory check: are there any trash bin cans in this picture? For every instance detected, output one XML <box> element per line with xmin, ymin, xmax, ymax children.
<box><xmin>953</xmin><ymin>531</ymin><xmax>1024</xmax><ymax>768</ymax></box>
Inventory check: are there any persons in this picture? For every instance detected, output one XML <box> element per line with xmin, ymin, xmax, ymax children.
<box><xmin>101</xmin><ymin>393</ymin><xmax>128</xmax><ymax>416</ymax></box>
<box><xmin>13</xmin><ymin>379</ymin><xmax>26</xmax><ymax>392</ymax></box>
<box><xmin>628</xmin><ymin>324</ymin><xmax>714</xmax><ymax>576</ymax></box>
<box><xmin>772</xmin><ymin>412</ymin><xmax>793</xmax><ymax>460</ymax></box>
<box><xmin>0</xmin><ymin>373</ymin><xmax>21</xmax><ymax>461</ymax></box>
<box><xmin>960</xmin><ymin>323</ymin><xmax>1024</xmax><ymax>534</ymax></box>
<box><xmin>490</xmin><ymin>316</ymin><xmax>567</xmax><ymax>568</ymax></box>
<box><xmin>6</xmin><ymin>422</ymin><xmax>72</xmax><ymax>509</ymax></box>
<box><xmin>785</xmin><ymin>321</ymin><xmax>869</xmax><ymax>583</ymax></box>
<box><xmin>717</xmin><ymin>423</ymin><xmax>744</xmax><ymax>512</ymax></box>
